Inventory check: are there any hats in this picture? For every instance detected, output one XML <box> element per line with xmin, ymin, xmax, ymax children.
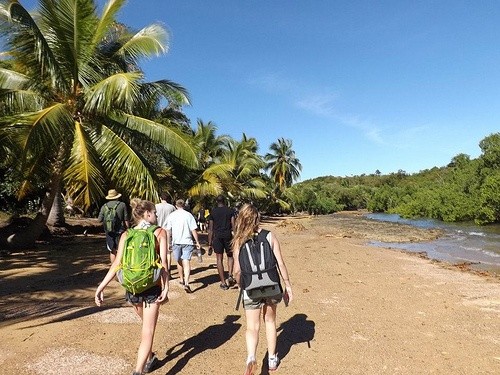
<box><xmin>105</xmin><ymin>189</ymin><xmax>122</xmax><ymax>200</ymax></box>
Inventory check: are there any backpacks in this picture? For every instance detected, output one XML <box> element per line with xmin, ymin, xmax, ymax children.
<box><xmin>115</xmin><ymin>224</ymin><xmax>161</xmax><ymax>294</ymax></box>
<box><xmin>104</xmin><ymin>201</ymin><xmax>122</xmax><ymax>233</ymax></box>
<box><xmin>239</xmin><ymin>229</ymin><xmax>283</xmax><ymax>305</ymax></box>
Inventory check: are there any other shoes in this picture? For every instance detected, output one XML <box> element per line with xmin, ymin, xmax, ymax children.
<box><xmin>226</xmin><ymin>276</ymin><xmax>234</xmax><ymax>287</ymax></box>
<box><xmin>220</xmin><ymin>283</ymin><xmax>228</xmax><ymax>290</ymax></box>
<box><xmin>184</xmin><ymin>285</ymin><xmax>192</xmax><ymax>293</ymax></box>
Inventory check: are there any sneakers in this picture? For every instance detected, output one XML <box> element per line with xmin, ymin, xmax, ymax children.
<box><xmin>244</xmin><ymin>355</ymin><xmax>258</xmax><ymax>375</ymax></box>
<box><xmin>267</xmin><ymin>353</ymin><xmax>280</xmax><ymax>370</ymax></box>
<box><xmin>143</xmin><ymin>352</ymin><xmax>163</xmax><ymax>372</ymax></box>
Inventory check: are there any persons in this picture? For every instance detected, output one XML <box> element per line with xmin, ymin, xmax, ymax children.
<box><xmin>230</xmin><ymin>204</ymin><xmax>293</xmax><ymax>375</ymax></box>
<box><xmin>94</xmin><ymin>200</ymin><xmax>167</xmax><ymax>375</ymax></box>
<box><xmin>99</xmin><ymin>189</ymin><xmax>238</xmax><ymax>289</ymax></box>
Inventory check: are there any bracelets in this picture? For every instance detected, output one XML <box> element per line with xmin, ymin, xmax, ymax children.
<box><xmin>283</xmin><ymin>279</ymin><xmax>289</xmax><ymax>282</ymax></box>
